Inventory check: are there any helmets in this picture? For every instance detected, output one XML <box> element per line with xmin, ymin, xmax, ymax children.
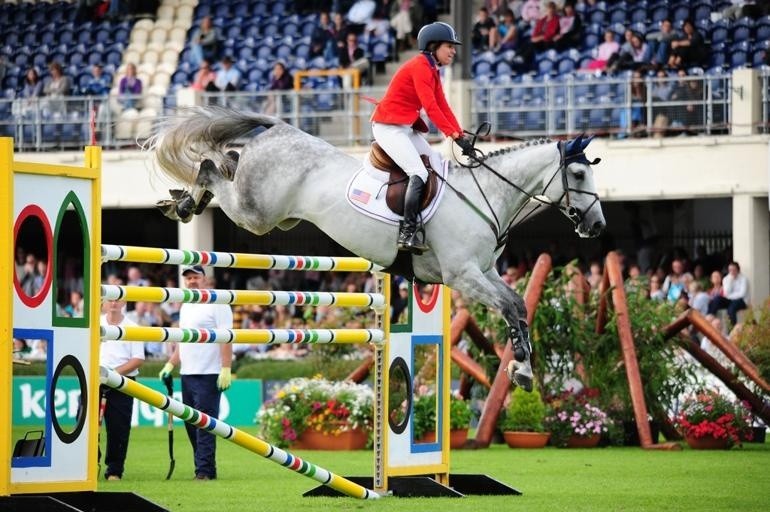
<box><xmin>417</xmin><ymin>22</ymin><xmax>464</xmax><ymax>53</ymax></box>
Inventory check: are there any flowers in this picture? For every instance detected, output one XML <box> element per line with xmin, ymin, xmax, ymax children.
<box><xmin>395</xmin><ymin>385</ymin><xmax>471</xmax><ymax>439</ymax></box>
<box><xmin>545</xmin><ymin>404</ymin><xmax>613</xmax><ymax>446</ymax></box>
<box><xmin>671</xmin><ymin>390</ymin><xmax>753</xmax><ymax>448</ymax></box>
<box><xmin>253</xmin><ymin>372</ymin><xmax>375</xmax><ymax>450</ymax></box>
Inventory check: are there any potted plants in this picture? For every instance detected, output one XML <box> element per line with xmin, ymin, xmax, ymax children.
<box><xmin>501</xmin><ymin>386</ymin><xmax>551</xmax><ymax>448</ymax></box>
<box><xmin>600</xmin><ymin>275</ymin><xmax>677</xmax><ymax>446</ymax></box>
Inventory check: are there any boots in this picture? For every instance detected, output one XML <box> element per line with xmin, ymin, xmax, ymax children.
<box><xmin>397</xmin><ymin>174</ymin><xmax>430</xmax><ymax>252</ymax></box>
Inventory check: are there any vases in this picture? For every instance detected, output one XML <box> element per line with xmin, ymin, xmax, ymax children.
<box><xmin>568</xmin><ymin>432</ymin><xmax>603</xmax><ymax>447</ymax></box>
<box><xmin>298</xmin><ymin>417</ymin><xmax>368</xmax><ymax>451</ymax></box>
<box><xmin>686</xmin><ymin>431</ymin><xmax>734</xmax><ymax>449</ymax></box>
<box><xmin>733</xmin><ymin>350</ymin><xmax>770</xmax><ymax>443</ymax></box>
<box><xmin>415</xmin><ymin>428</ymin><xmax>469</xmax><ymax>449</ymax></box>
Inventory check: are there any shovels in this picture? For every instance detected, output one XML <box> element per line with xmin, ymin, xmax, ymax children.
<box><xmin>164</xmin><ymin>375</ymin><xmax>175</xmax><ymax>480</ymax></box>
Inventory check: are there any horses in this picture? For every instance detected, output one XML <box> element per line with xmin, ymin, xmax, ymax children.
<box><xmin>135</xmin><ymin>107</ymin><xmax>607</xmax><ymax>394</ymax></box>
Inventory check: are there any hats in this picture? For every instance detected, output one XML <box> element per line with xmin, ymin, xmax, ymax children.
<box><xmin>182</xmin><ymin>265</ymin><xmax>206</xmax><ymax>277</ymax></box>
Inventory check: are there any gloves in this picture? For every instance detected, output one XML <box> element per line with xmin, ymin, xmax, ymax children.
<box><xmin>216</xmin><ymin>367</ymin><xmax>232</xmax><ymax>392</ymax></box>
<box><xmin>158</xmin><ymin>362</ymin><xmax>175</xmax><ymax>380</ymax></box>
<box><xmin>456</xmin><ymin>135</ymin><xmax>476</xmax><ymax>155</ymax></box>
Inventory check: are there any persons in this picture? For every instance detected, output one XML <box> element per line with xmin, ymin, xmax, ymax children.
<box><xmin>503</xmin><ymin>258</ymin><xmax>752</xmax><ymax>363</ymax></box>
<box><xmin>14</xmin><ymin>249</ymin><xmax>180</xmax><ymax>359</ymax></box>
<box><xmin>158</xmin><ymin>265</ymin><xmax>236</xmax><ymax>480</ymax></box>
<box><xmin>186</xmin><ymin>0</ymin><xmax>423</xmax><ymax>114</ymax></box>
<box><xmin>231</xmin><ymin>274</ymin><xmax>409</xmax><ymax>359</ymax></box>
<box><xmin>89</xmin><ymin>2</ymin><xmax>130</xmax><ymax>18</ymax></box>
<box><xmin>368</xmin><ymin>20</ymin><xmax>475</xmax><ymax>250</ymax></box>
<box><xmin>98</xmin><ymin>298</ymin><xmax>147</xmax><ymax>481</ymax></box>
<box><xmin>473</xmin><ymin>0</ymin><xmax>769</xmax><ymax>142</ymax></box>
<box><xmin>0</xmin><ymin>60</ymin><xmax>143</xmax><ymax>113</ymax></box>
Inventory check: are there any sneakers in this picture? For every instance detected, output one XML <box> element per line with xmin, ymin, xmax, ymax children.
<box><xmin>107</xmin><ymin>474</ymin><xmax>122</xmax><ymax>481</ymax></box>
<box><xmin>192</xmin><ymin>473</ymin><xmax>217</xmax><ymax>481</ymax></box>
